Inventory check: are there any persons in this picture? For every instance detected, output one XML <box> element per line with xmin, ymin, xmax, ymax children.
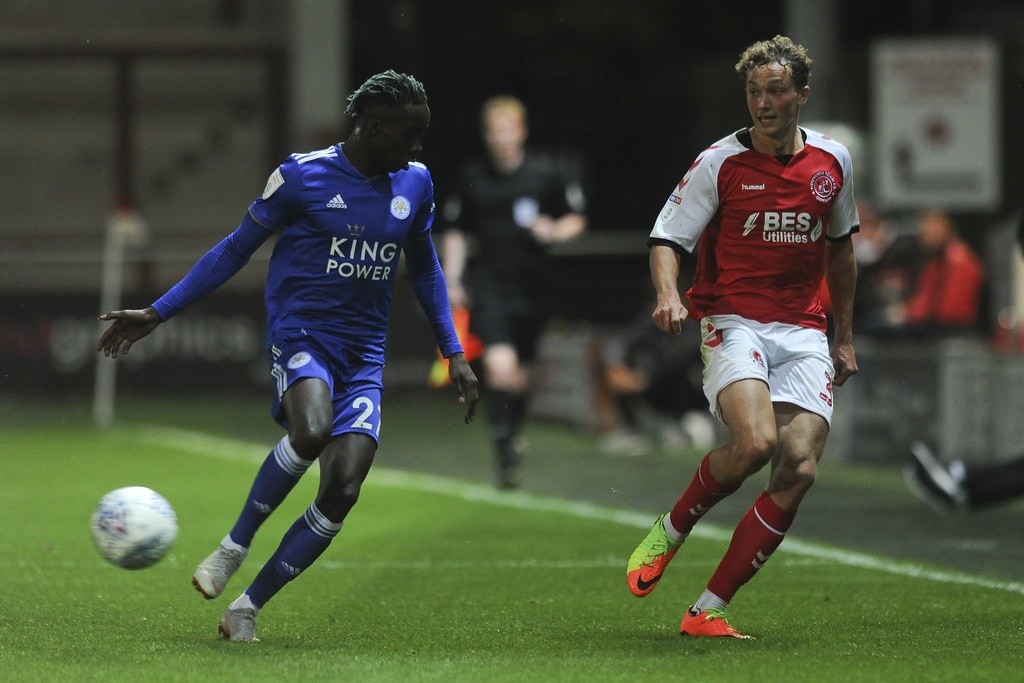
<box><xmin>853</xmin><ymin>210</ymin><xmax>981</xmax><ymax>326</ymax></box>
<box><xmin>95</xmin><ymin>69</ymin><xmax>478</xmax><ymax>641</ymax></box>
<box><xmin>443</xmin><ymin>97</ymin><xmax>586</xmax><ymax>490</ymax></box>
<box><xmin>628</xmin><ymin>36</ymin><xmax>859</xmax><ymax>637</ymax></box>
<box><xmin>917</xmin><ymin>457</ymin><xmax>1024</xmax><ymax>513</ymax></box>
<box><xmin>596</xmin><ymin>303</ymin><xmax>716</xmax><ymax>458</ymax></box>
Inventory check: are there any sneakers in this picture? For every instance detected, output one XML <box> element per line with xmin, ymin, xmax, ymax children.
<box><xmin>191</xmin><ymin>545</ymin><xmax>245</xmax><ymax>601</ymax></box>
<box><xmin>627</xmin><ymin>512</ymin><xmax>685</xmax><ymax>598</ymax></box>
<box><xmin>218</xmin><ymin>602</ymin><xmax>261</xmax><ymax>644</ymax></box>
<box><xmin>680</xmin><ymin>605</ymin><xmax>757</xmax><ymax>640</ymax></box>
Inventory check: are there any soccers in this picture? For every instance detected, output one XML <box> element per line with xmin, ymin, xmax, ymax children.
<box><xmin>90</xmin><ymin>487</ymin><xmax>181</xmax><ymax>571</ymax></box>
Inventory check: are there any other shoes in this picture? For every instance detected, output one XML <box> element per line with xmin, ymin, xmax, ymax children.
<box><xmin>908</xmin><ymin>439</ymin><xmax>969</xmax><ymax>519</ymax></box>
<box><xmin>491</xmin><ymin>432</ymin><xmax>527</xmax><ymax>460</ymax></box>
<box><xmin>495</xmin><ymin>462</ymin><xmax>523</xmax><ymax>491</ymax></box>
